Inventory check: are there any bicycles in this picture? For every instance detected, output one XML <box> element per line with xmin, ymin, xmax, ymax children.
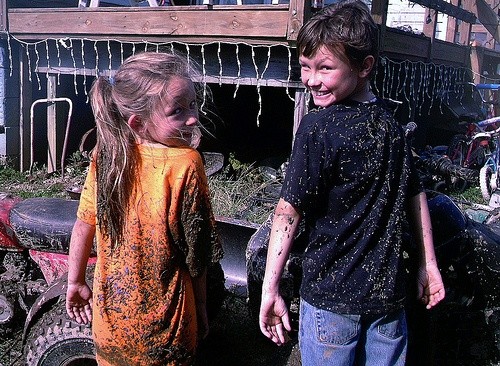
<box><xmin>479</xmin><ymin>116</ymin><xmax>500</xmax><ymax>203</ymax></box>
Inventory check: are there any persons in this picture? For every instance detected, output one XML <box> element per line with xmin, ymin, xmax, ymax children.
<box><xmin>66</xmin><ymin>51</ymin><xmax>225</xmax><ymax>365</ymax></box>
<box><xmin>257</xmin><ymin>1</ymin><xmax>449</xmax><ymax>366</ymax></box>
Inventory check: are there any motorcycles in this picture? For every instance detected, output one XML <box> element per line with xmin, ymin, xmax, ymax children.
<box><xmin>1</xmin><ymin>186</ymin><xmax>500</xmax><ymax>366</ymax></box>
